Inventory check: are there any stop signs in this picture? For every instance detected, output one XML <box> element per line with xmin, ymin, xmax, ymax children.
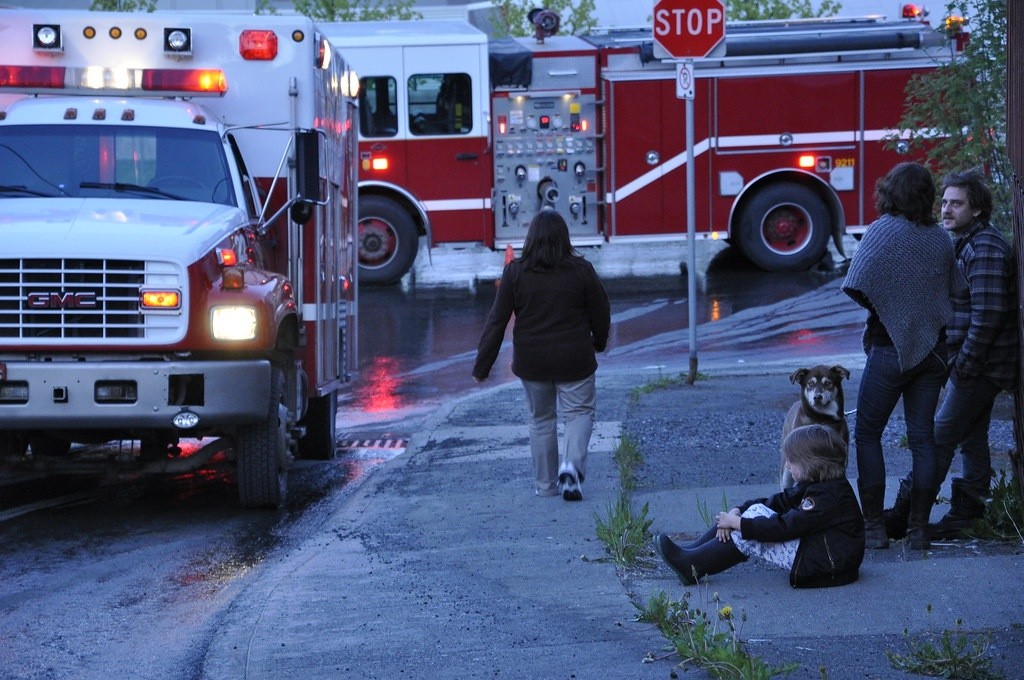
<box><xmin>651</xmin><ymin>0</ymin><xmax>727</xmax><ymax>59</ymax></box>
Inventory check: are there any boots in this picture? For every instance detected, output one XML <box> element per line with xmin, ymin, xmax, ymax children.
<box><xmin>857</xmin><ymin>471</ymin><xmax>890</xmax><ymax>549</ymax></box>
<box><xmin>882</xmin><ymin>471</ymin><xmax>941</xmax><ymax>540</ymax></box>
<box><xmin>926</xmin><ymin>477</ymin><xmax>992</xmax><ymax>540</ymax></box>
<box><xmin>907</xmin><ymin>471</ymin><xmax>941</xmax><ymax>551</ymax></box>
<box><xmin>657</xmin><ymin>533</ymin><xmax>749</xmax><ymax>587</ymax></box>
<box><xmin>654</xmin><ymin>520</ymin><xmax>718</xmax><ymax>555</ymax></box>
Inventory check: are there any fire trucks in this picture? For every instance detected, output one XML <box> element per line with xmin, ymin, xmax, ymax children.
<box><xmin>0</xmin><ymin>4</ymin><xmax>360</xmax><ymax>507</ymax></box>
<box><xmin>318</xmin><ymin>7</ymin><xmax>996</xmax><ymax>285</ymax></box>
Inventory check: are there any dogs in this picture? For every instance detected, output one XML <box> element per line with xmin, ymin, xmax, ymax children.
<box><xmin>779</xmin><ymin>364</ymin><xmax>851</xmax><ymax>493</ymax></box>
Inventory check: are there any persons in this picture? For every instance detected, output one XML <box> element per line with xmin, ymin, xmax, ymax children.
<box><xmin>653</xmin><ymin>425</ymin><xmax>866</xmax><ymax>588</ymax></box>
<box><xmin>841</xmin><ymin>163</ymin><xmax>967</xmax><ymax>549</ymax></box>
<box><xmin>884</xmin><ymin>169</ymin><xmax>1018</xmax><ymax>542</ymax></box>
<box><xmin>472</xmin><ymin>210</ymin><xmax>610</xmax><ymax>502</ymax></box>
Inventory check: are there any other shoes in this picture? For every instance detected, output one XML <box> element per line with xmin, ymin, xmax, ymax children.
<box><xmin>535</xmin><ymin>488</ymin><xmax>559</xmax><ymax>497</ymax></box>
<box><xmin>558</xmin><ymin>461</ymin><xmax>584</xmax><ymax>501</ymax></box>
